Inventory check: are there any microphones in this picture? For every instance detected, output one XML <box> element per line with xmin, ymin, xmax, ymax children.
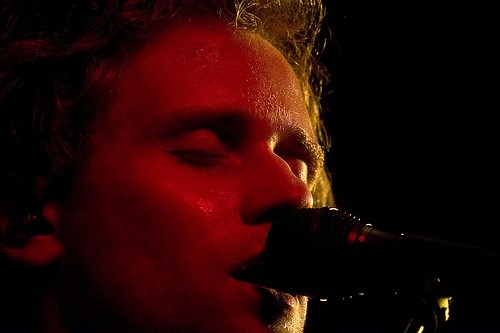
<box><xmin>259</xmin><ymin>207</ymin><xmax>453</xmax><ymax>301</ymax></box>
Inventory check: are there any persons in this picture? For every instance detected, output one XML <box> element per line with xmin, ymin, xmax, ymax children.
<box><xmin>0</xmin><ymin>0</ymin><xmax>331</xmax><ymax>333</ymax></box>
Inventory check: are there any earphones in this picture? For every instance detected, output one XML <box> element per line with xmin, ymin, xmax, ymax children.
<box><xmin>21</xmin><ymin>211</ymin><xmax>41</xmax><ymax>229</ymax></box>
